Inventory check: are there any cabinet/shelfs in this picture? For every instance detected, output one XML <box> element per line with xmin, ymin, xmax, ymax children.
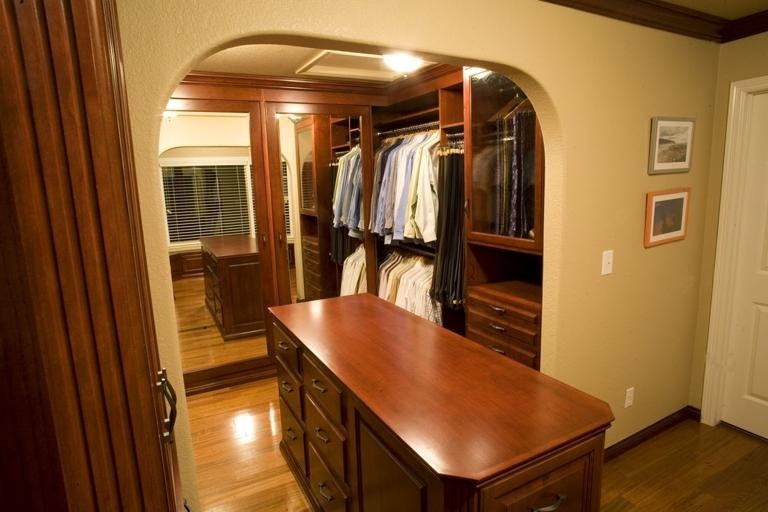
<box><xmin>195</xmin><ymin>235</ymin><xmax>267</xmax><ymax>343</ymax></box>
<box><xmin>294</xmin><ymin>114</ymin><xmax>365</xmax><ymax>301</ymax></box>
<box><xmin>266</xmin><ymin>294</ymin><xmax>616</xmax><ymax>511</ymax></box>
<box><xmin>370</xmin><ymin>62</ymin><xmax>540</xmax><ymax>372</ymax></box>
<box><xmin>3</xmin><ymin>1</ymin><xmax>186</xmax><ymax>512</ymax></box>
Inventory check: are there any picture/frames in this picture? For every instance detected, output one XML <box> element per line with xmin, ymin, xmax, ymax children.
<box><xmin>643</xmin><ymin>116</ymin><xmax>696</xmax><ymax>249</ymax></box>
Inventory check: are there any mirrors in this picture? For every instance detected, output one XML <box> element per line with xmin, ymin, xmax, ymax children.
<box><xmin>160</xmin><ymin>110</ymin><xmax>370</xmax><ymax>377</ymax></box>
